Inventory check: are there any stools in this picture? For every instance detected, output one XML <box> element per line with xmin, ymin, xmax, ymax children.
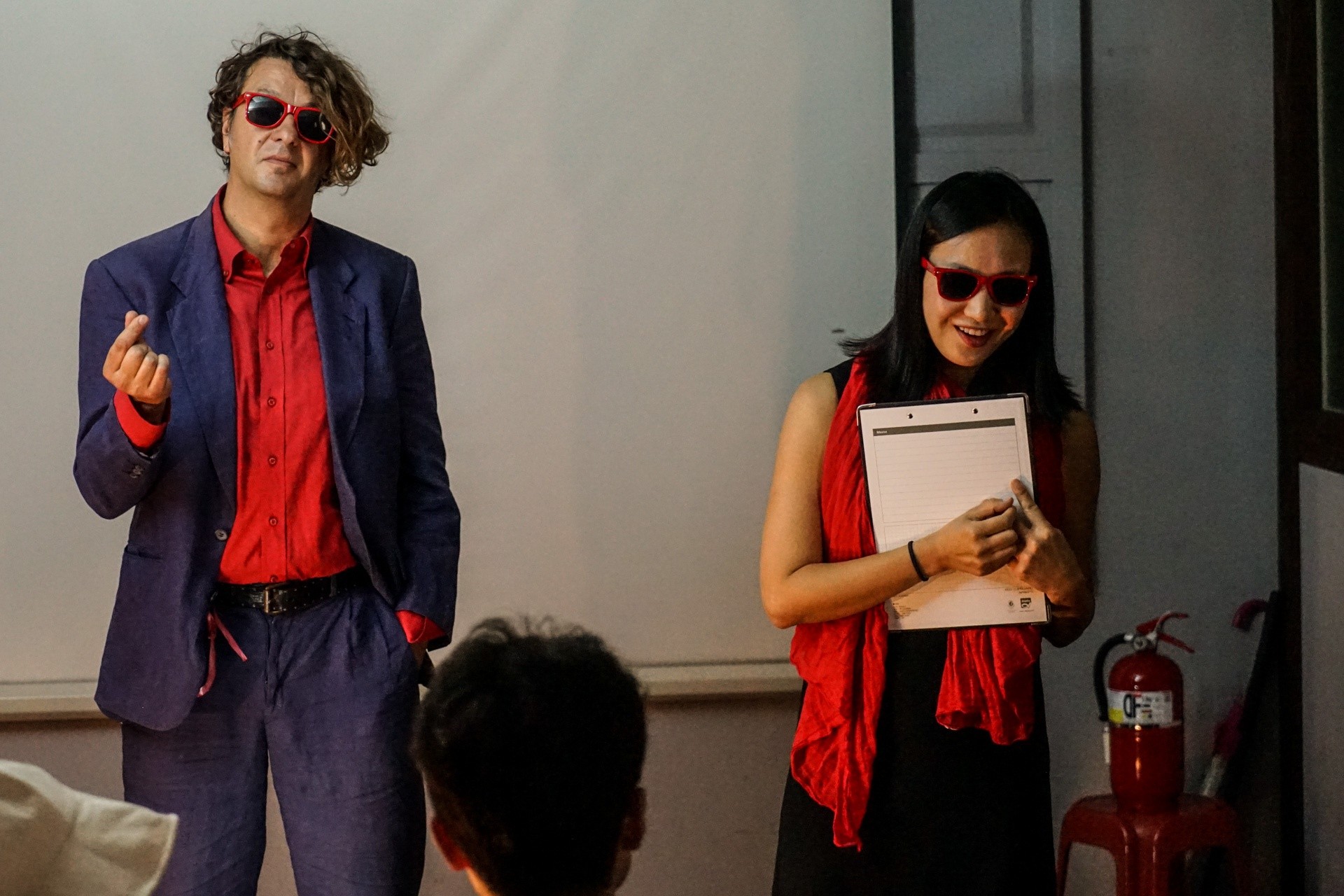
<box><xmin>1056</xmin><ymin>792</ymin><xmax>1252</xmax><ymax>896</ymax></box>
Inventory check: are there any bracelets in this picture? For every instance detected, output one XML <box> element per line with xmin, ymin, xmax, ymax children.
<box><xmin>908</xmin><ymin>540</ymin><xmax>929</xmax><ymax>582</ymax></box>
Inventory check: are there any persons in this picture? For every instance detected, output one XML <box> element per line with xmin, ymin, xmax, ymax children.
<box><xmin>759</xmin><ymin>170</ymin><xmax>1104</xmax><ymax>896</ymax></box>
<box><xmin>414</xmin><ymin>613</ymin><xmax>650</xmax><ymax>895</ymax></box>
<box><xmin>74</xmin><ymin>26</ymin><xmax>460</xmax><ymax>896</ymax></box>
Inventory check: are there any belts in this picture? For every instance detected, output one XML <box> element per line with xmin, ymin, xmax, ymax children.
<box><xmin>211</xmin><ymin>566</ymin><xmax>365</xmax><ymax>613</ymax></box>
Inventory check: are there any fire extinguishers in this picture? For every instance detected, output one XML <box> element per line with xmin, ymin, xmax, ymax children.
<box><xmin>1103</xmin><ymin>609</ymin><xmax>1199</xmax><ymax>807</ymax></box>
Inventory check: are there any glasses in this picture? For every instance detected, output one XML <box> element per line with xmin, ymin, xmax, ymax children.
<box><xmin>920</xmin><ymin>257</ymin><xmax>1037</xmax><ymax>308</ymax></box>
<box><xmin>233</xmin><ymin>91</ymin><xmax>335</xmax><ymax>144</ymax></box>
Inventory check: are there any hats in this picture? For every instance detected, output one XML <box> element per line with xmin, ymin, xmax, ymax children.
<box><xmin>1</xmin><ymin>762</ymin><xmax>180</xmax><ymax>896</ymax></box>
